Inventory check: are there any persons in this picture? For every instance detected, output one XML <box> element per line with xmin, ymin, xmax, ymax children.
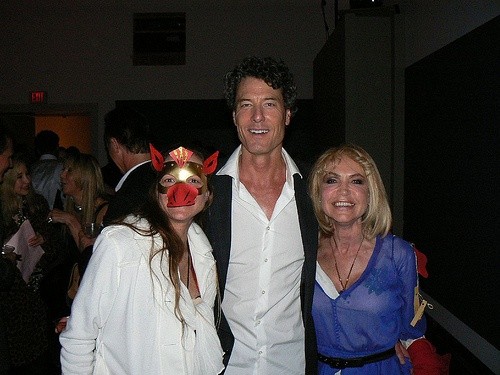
<box><xmin>0</xmin><ymin>108</ymin><xmax>450</xmax><ymax>375</ymax></box>
<box><xmin>193</xmin><ymin>57</ymin><xmax>410</xmax><ymax>375</ymax></box>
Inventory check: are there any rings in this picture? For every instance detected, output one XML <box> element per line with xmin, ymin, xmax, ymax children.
<box><xmin>48</xmin><ymin>217</ymin><xmax>54</xmax><ymax>223</ymax></box>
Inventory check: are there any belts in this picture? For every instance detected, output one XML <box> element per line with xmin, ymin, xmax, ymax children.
<box><xmin>318</xmin><ymin>345</ymin><xmax>396</xmax><ymax>369</ymax></box>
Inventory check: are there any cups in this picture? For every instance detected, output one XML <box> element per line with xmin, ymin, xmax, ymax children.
<box><xmin>83</xmin><ymin>223</ymin><xmax>101</xmax><ymax>238</ymax></box>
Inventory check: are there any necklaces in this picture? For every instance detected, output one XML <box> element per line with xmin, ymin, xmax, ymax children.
<box><xmin>74</xmin><ymin>204</ymin><xmax>86</xmax><ymax>212</ymax></box>
<box><xmin>329</xmin><ymin>233</ymin><xmax>366</xmax><ymax>304</ymax></box>
<box><xmin>187</xmin><ymin>253</ymin><xmax>191</xmax><ymax>290</ymax></box>
